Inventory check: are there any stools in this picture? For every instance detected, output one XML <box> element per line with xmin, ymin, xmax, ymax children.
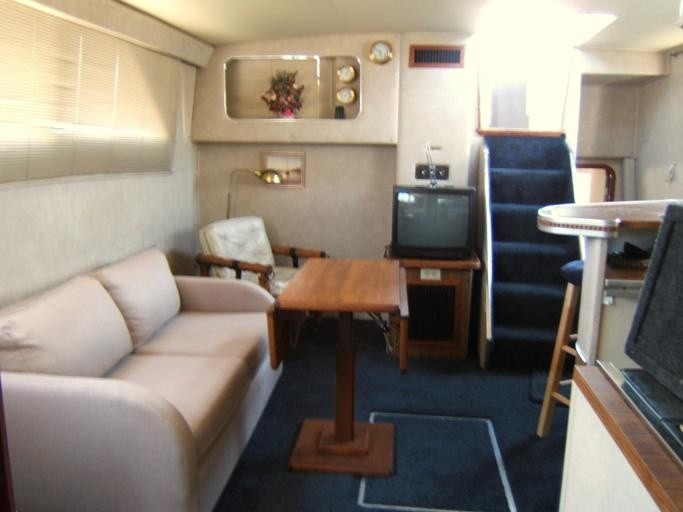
<box><xmin>536</xmin><ymin>260</ymin><xmax>584</xmax><ymax>440</ymax></box>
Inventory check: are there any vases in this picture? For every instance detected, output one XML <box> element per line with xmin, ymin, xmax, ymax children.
<box><xmin>277</xmin><ymin>107</ymin><xmax>295</xmax><ymax>118</ymax></box>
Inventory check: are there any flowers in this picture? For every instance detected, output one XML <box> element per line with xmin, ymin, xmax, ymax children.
<box><xmin>261</xmin><ymin>68</ymin><xmax>305</xmax><ymax>114</ymax></box>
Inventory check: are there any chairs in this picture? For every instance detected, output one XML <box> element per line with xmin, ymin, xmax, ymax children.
<box><xmin>195</xmin><ymin>214</ymin><xmax>330</xmax><ymax>371</ymax></box>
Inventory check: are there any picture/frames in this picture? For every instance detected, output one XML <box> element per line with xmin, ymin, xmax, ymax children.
<box><xmin>261</xmin><ymin>151</ymin><xmax>305</xmax><ymax>190</ymax></box>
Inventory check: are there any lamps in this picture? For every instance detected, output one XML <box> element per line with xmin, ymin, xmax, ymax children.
<box><xmin>226</xmin><ymin>165</ymin><xmax>281</xmax><ymax>219</ymax></box>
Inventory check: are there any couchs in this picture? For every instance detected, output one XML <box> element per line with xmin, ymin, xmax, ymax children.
<box><xmin>0</xmin><ymin>245</ymin><xmax>281</xmax><ymax>512</ymax></box>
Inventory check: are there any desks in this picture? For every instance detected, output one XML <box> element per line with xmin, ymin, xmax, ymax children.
<box><xmin>267</xmin><ymin>255</ymin><xmax>410</xmax><ymax>478</ymax></box>
<box><xmin>557</xmin><ymin>362</ymin><xmax>683</xmax><ymax>511</ymax></box>
<box><xmin>387</xmin><ymin>248</ymin><xmax>481</xmax><ymax>362</ymax></box>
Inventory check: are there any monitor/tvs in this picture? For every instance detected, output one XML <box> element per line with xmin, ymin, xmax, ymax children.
<box><xmin>618</xmin><ymin>202</ymin><xmax>683</xmax><ymax>462</ymax></box>
<box><xmin>390</xmin><ymin>183</ymin><xmax>478</xmax><ymax>261</ymax></box>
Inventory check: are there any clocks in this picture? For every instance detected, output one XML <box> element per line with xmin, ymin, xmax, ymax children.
<box><xmin>368</xmin><ymin>40</ymin><xmax>393</xmax><ymax>66</ymax></box>
<box><xmin>336</xmin><ymin>88</ymin><xmax>356</xmax><ymax>106</ymax></box>
<box><xmin>336</xmin><ymin>65</ymin><xmax>356</xmax><ymax>83</ymax></box>
